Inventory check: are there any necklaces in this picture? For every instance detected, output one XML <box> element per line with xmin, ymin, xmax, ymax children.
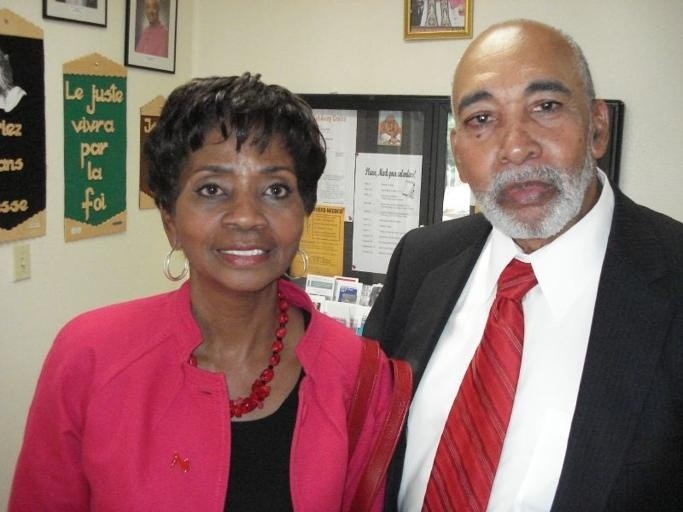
<box><xmin>187</xmin><ymin>293</ymin><xmax>290</xmax><ymax>418</ymax></box>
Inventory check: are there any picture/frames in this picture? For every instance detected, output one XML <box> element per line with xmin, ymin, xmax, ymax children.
<box><xmin>123</xmin><ymin>0</ymin><xmax>177</xmax><ymax>75</ymax></box>
<box><xmin>402</xmin><ymin>0</ymin><xmax>473</xmax><ymax>41</ymax></box>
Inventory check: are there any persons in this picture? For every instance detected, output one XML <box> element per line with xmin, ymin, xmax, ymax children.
<box><xmin>360</xmin><ymin>16</ymin><xmax>683</xmax><ymax>511</ymax></box>
<box><xmin>6</xmin><ymin>71</ymin><xmax>395</xmax><ymax>511</ymax></box>
<box><xmin>136</xmin><ymin>0</ymin><xmax>168</xmax><ymax>57</ymax></box>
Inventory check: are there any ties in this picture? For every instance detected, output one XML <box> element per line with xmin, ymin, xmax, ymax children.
<box><xmin>420</xmin><ymin>257</ymin><xmax>540</xmax><ymax>512</ymax></box>
